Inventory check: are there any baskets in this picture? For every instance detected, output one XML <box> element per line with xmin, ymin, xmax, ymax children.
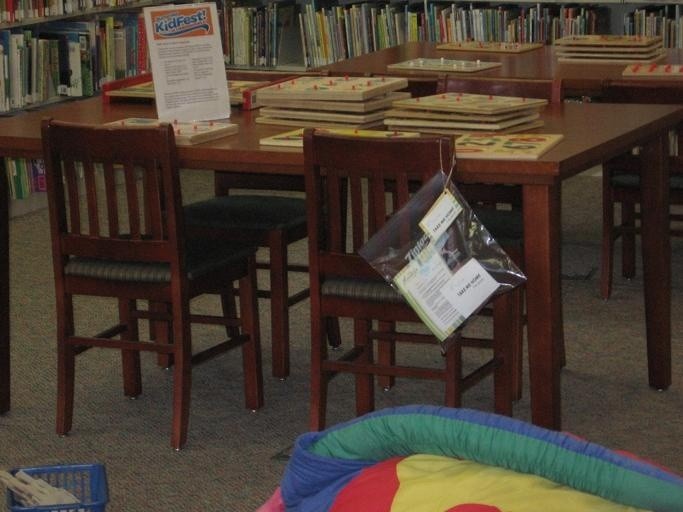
<box><xmin>7</xmin><ymin>465</ymin><xmax>108</xmax><ymax>512</ymax></box>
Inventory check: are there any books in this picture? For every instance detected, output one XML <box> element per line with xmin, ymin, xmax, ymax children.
<box><xmin>1</xmin><ymin>1</ymin><xmax>153</xmax><ymax>113</ymax></box>
<box><xmin>4</xmin><ymin>157</ymin><xmax>104</xmax><ymax>201</ymax></box>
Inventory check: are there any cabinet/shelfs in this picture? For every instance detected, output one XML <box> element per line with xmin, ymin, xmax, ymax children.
<box><xmin>0</xmin><ymin>0</ymin><xmax>683</xmax><ymax>215</ymax></box>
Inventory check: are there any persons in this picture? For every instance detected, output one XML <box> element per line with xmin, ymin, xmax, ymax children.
<box><xmin>218</xmin><ymin>1</ymin><xmax>683</xmax><ymax>70</ymax></box>
